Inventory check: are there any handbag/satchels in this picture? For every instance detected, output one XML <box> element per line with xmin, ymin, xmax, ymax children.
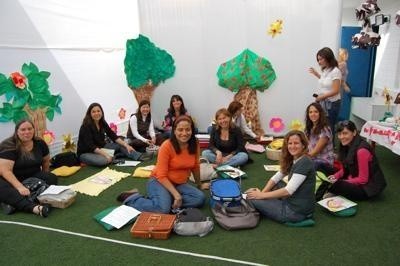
<box><xmin>49</xmin><ymin>151</ymin><xmax>81</xmax><ymax>168</ymax></box>
<box><xmin>189</xmin><ymin>157</ymin><xmax>218</xmax><ymax>182</ymax></box>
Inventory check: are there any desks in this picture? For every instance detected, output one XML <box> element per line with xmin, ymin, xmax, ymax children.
<box><xmin>359</xmin><ymin>118</ymin><xmax>400</xmax><ymax>157</ymax></box>
<box><xmin>349</xmin><ymin>95</ymin><xmax>400</xmax><ymax>134</ymax></box>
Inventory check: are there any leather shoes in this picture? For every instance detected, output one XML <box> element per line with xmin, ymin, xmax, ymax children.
<box><xmin>116</xmin><ymin>188</ymin><xmax>138</xmax><ymax>202</ymax></box>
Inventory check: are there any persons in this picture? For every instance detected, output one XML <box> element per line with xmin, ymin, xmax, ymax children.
<box><xmin>76</xmin><ymin>102</ymin><xmax>152</xmax><ymax>166</ymax></box>
<box><xmin>336</xmin><ymin>47</ymin><xmax>352</xmax><ymax>94</ymax></box>
<box><xmin>128</xmin><ymin>98</ymin><xmax>165</xmax><ymax>153</ymax></box>
<box><xmin>115</xmin><ymin>115</ymin><xmax>212</xmax><ymax>217</ymax></box>
<box><xmin>0</xmin><ymin>118</ymin><xmax>57</xmax><ymax>218</ymax></box>
<box><xmin>158</xmin><ymin>93</ymin><xmax>388</xmax><ymax>225</ymax></box>
<box><xmin>306</xmin><ymin>46</ymin><xmax>344</xmax><ymax>153</ymax></box>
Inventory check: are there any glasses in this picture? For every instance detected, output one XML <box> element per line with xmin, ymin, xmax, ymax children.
<box><xmin>336</xmin><ymin>120</ymin><xmax>350</xmax><ymax>127</ymax></box>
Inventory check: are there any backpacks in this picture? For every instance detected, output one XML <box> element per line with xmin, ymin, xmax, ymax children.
<box><xmin>173</xmin><ymin>208</ymin><xmax>215</xmax><ymax>238</ymax></box>
<box><xmin>211</xmin><ymin>199</ymin><xmax>260</xmax><ymax>231</ymax></box>
<box><xmin>21</xmin><ymin>177</ymin><xmax>49</xmax><ymax>203</ymax></box>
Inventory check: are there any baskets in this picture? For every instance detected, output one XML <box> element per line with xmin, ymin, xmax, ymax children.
<box><xmin>265</xmin><ymin>146</ymin><xmax>282</xmax><ymax>161</ymax></box>
